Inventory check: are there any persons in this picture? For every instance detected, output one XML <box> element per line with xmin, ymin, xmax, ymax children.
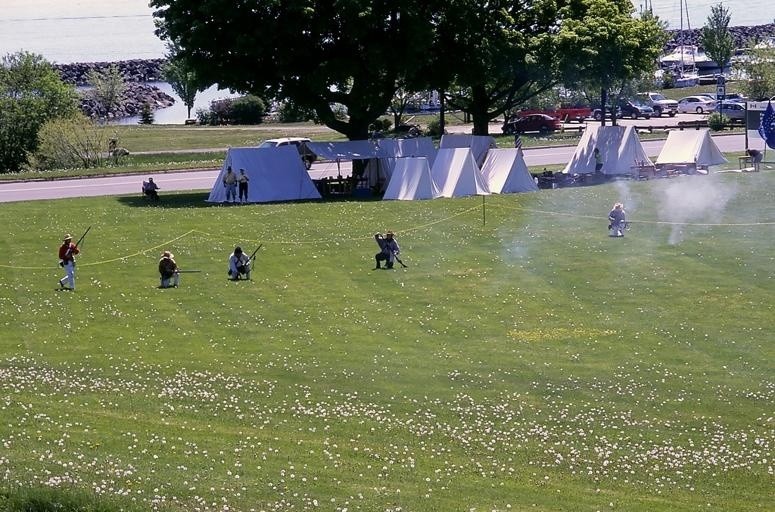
<box><xmin>142</xmin><ymin>178</ymin><xmax>160</xmax><ymax>199</ymax></box>
<box><xmin>222</xmin><ymin>165</ymin><xmax>238</xmax><ymax>205</ymax></box>
<box><xmin>159</xmin><ymin>251</ymin><xmax>179</xmax><ymax>289</ymax></box>
<box><xmin>746</xmin><ymin>147</ymin><xmax>764</xmax><ymax>171</ymax></box>
<box><xmin>235</xmin><ymin>168</ymin><xmax>249</xmax><ymax>205</ymax></box>
<box><xmin>372</xmin><ymin>231</ymin><xmax>399</xmax><ymax>269</ymax></box>
<box><xmin>592</xmin><ymin>147</ymin><xmax>604</xmax><ymax>174</ymax></box>
<box><xmin>59</xmin><ymin>233</ymin><xmax>80</xmax><ymax>291</ymax></box>
<box><xmin>228</xmin><ymin>246</ymin><xmax>251</xmax><ymax>280</ymax></box>
<box><xmin>608</xmin><ymin>202</ymin><xmax>626</xmax><ymax>237</ymax></box>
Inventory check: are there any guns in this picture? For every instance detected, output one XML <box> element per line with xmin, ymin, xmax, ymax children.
<box><xmin>244</xmin><ymin>244</ymin><xmax>263</xmax><ymax>266</ymax></box>
<box><xmin>172</xmin><ymin>270</ymin><xmax>201</xmax><ymax>272</ymax></box>
<box><xmin>60</xmin><ymin>226</ymin><xmax>91</xmax><ymax>267</ymax></box>
<box><xmin>380</xmin><ymin>234</ymin><xmax>408</xmax><ymax>268</ymax></box>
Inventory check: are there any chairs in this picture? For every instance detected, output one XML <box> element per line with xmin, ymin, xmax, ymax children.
<box><xmin>369</xmin><ymin>177</ymin><xmax>387</xmax><ymax>199</ymax></box>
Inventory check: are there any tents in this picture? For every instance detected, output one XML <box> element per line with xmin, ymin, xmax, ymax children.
<box><xmin>204</xmin><ymin>144</ymin><xmax>323</xmax><ymax>205</ymax></box>
<box><xmin>654</xmin><ymin>129</ymin><xmax>729</xmax><ymax>168</ymax></box>
<box><xmin>357</xmin><ymin>135</ymin><xmax>540</xmax><ymax>199</ymax></box>
<box><xmin>563</xmin><ymin>124</ymin><xmax>654</xmax><ymax>174</ymax></box>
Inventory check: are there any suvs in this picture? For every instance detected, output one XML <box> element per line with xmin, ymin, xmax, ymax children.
<box><xmin>257</xmin><ymin>136</ymin><xmax>317</xmax><ymax>171</ymax></box>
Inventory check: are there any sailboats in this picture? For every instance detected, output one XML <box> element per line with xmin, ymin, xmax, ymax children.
<box><xmin>638</xmin><ymin>0</ymin><xmax>734</xmax><ymax>90</ymax></box>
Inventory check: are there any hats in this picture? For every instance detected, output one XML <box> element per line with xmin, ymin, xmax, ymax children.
<box><xmin>613</xmin><ymin>202</ymin><xmax>624</xmax><ymax>211</ymax></box>
<box><xmin>161</xmin><ymin>251</ymin><xmax>174</xmax><ymax>259</ymax></box>
<box><xmin>385</xmin><ymin>230</ymin><xmax>394</xmax><ymax>235</ymax></box>
<box><xmin>234</xmin><ymin>247</ymin><xmax>242</xmax><ymax>254</ymax></box>
<box><xmin>62</xmin><ymin>233</ymin><xmax>73</xmax><ymax>241</ymax></box>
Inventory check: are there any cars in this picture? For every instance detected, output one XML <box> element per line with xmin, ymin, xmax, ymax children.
<box><xmin>501</xmin><ymin>91</ymin><xmax>775</xmax><ymax>134</ymax></box>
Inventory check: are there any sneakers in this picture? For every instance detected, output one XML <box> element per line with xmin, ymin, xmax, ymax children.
<box><xmin>174</xmin><ymin>284</ymin><xmax>178</xmax><ymax>289</ymax></box>
<box><xmin>60</xmin><ymin>280</ymin><xmax>74</xmax><ymax>290</ymax></box>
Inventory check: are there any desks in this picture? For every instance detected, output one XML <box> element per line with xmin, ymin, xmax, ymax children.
<box><xmin>537</xmin><ymin>155</ymin><xmax>760</xmax><ymax>189</ymax></box>
<box><xmin>312</xmin><ymin>178</ymin><xmax>369</xmax><ymax>202</ymax></box>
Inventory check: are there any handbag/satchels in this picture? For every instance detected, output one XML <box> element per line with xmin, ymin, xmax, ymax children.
<box><xmin>71</xmin><ymin>256</ymin><xmax>77</xmax><ymax>267</ymax></box>
<box><xmin>379</xmin><ymin>252</ymin><xmax>386</xmax><ymax>261</ymax></box>
<box><xmin>227</xmin><ymin>268</ymin><xmax>232</xmax><ymax>276</ymax></box>
